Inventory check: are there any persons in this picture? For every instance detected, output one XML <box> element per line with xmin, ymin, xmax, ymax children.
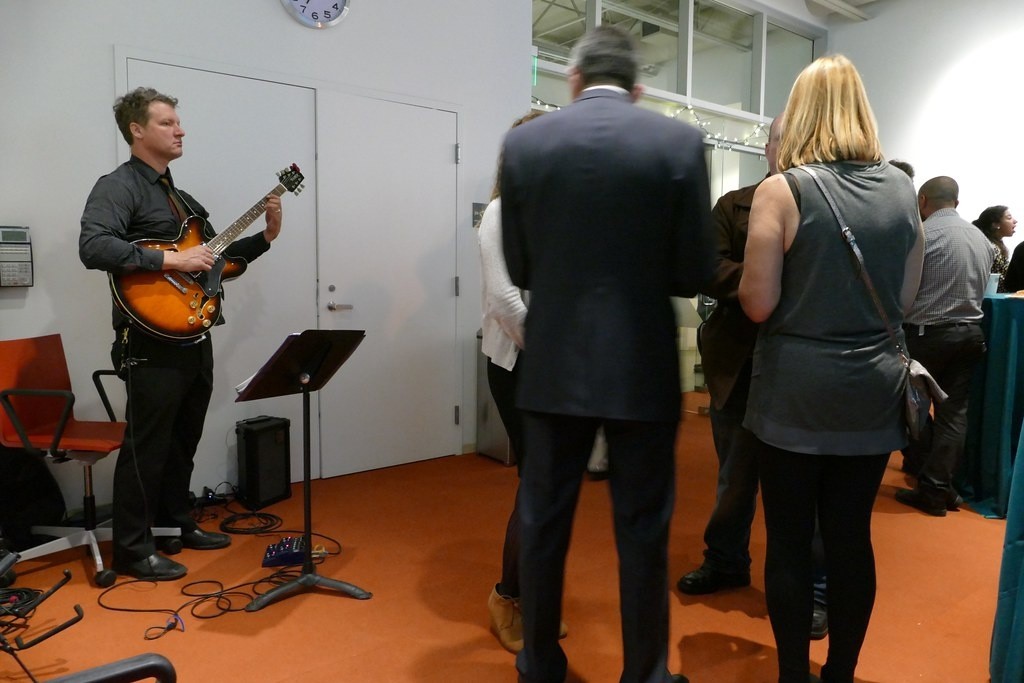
<box><xmin>736</xmin><ymin>55</ymin><xmax>926</xmax><ymax>683</ymax></box>
<box><xmin>501</xmin><ymin>25</ymin><xmax>716</xmax><ymax>683</ymax></box>
<box><xmin>79</xmin><ymin>87</ymin><xmax>282</xmax><ymax>581</ymax></box>
<box><xmin>888</xmin><ymin>161</ymin><xmax>1024</xmax><ymax>518</ymax></box>
<box><xmin>478</xmin><ymin>111</ymin><xmax>567</xmax><ymax>652</ymax></box>
<box><xmin>677</xmin><ymin>113</ymin><xmax>827</xmax><ymax>640</ymax></box>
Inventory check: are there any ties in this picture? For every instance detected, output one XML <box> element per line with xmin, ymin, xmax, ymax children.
<box><xmin>157</xmin><ymin>178</ymin><xmax>188</xmax><ymax>225</ymax></box>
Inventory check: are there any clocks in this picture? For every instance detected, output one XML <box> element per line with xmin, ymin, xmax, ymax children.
<box><xmin>280</xmin><ymin>0</ymin><xmax>351</xmax><ymax>30</ymax></box>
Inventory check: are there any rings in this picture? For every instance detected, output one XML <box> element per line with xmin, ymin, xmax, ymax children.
<box><xmin>277</xmin><ymin>210</ymin><xmax>280</xmax><ymax>213</ymax></box>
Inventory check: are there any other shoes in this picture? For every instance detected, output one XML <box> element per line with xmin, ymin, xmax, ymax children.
<box><xmin>902</xmin><ymin>458</ymin><xmax>925</xmax><ymax>476</ymax></box>
<box><xmin>895</xmin><ymin>487</ymin><xmax>963</xmax><ymax>515</ymax></box>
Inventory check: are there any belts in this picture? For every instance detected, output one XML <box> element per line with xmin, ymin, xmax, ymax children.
<box><xmin>902</xmin><ymin>322</ymin><xmax>967</xmax><ymax>334</ymax></box>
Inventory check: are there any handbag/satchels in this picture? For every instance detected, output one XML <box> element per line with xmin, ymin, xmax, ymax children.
<box><xmin>906</xmin><ymin>360</ymin><xmax>948</xmax><ymax>442</ymax></box>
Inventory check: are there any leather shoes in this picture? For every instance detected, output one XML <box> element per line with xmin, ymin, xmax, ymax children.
<box><xmin>671</xmin><ymin>674</ymin><xmax>690</xmax><ymax>683</ymax></box>
<box><xmin>111</xmin><ymin>551</ymin><xmax>189</xmax><ymax>582</ymax></box>
<box><xmin>515</xmin><ymin>646</ymin><xmax>526</xmax><ymax>683</ymax></box>
<box><xmin>811</xmin><ymin>601</ymin><xmax>828</xmax><ymax>639</ymax></box>
<box><xmin>678</xmin><ymin>559</ymin><xmax>751</xmax><ymax>595</ymax></box>
<box><xmin>179</xmin><ymin>527</ymin><xmax>231</xmax><ymax>550</ymax></box>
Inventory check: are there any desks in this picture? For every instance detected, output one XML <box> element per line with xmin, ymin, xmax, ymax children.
<box><xmin>964</xmin><ymin>295</ymin><xmax>1024</xmax><ymax>518</ymax></box>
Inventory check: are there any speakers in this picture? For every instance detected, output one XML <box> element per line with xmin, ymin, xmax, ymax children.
<box><xmin>235</xmin><ymin>415</ymin><xmax>292</xmax><ymax>512</ymax></box>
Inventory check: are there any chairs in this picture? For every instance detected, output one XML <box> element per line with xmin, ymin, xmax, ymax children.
<box><xmin>0</xmin><ymin>333</ymin><xmax>183</xmax><ymax>588</ymax></box>
<box><xmin>45</xmin><ymin>651</ymin><xmax>176</xmax><ymax>683</ymax></box>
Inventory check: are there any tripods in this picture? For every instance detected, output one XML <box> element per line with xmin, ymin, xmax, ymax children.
<box><xmin>234</xmin><ymin>330</ymin><xmax>373</xmax><ymax>612</ymax></box>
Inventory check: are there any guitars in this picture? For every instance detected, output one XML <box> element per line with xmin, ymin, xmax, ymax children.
<box><xmin>107</xmin><ymin>162</ymin><xmax>305</xmax><ymax>346</ymax></box>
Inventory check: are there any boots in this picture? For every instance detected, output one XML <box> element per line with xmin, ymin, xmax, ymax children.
<box><xmin>488</xmin><ymin>583</ymin><xmax>524</xmax><ymax>654</ymax></box>
<box><xmin>559</xmin><ymin>623</ymin><xmax>568</xmax><ymax>638</ymax></box>
